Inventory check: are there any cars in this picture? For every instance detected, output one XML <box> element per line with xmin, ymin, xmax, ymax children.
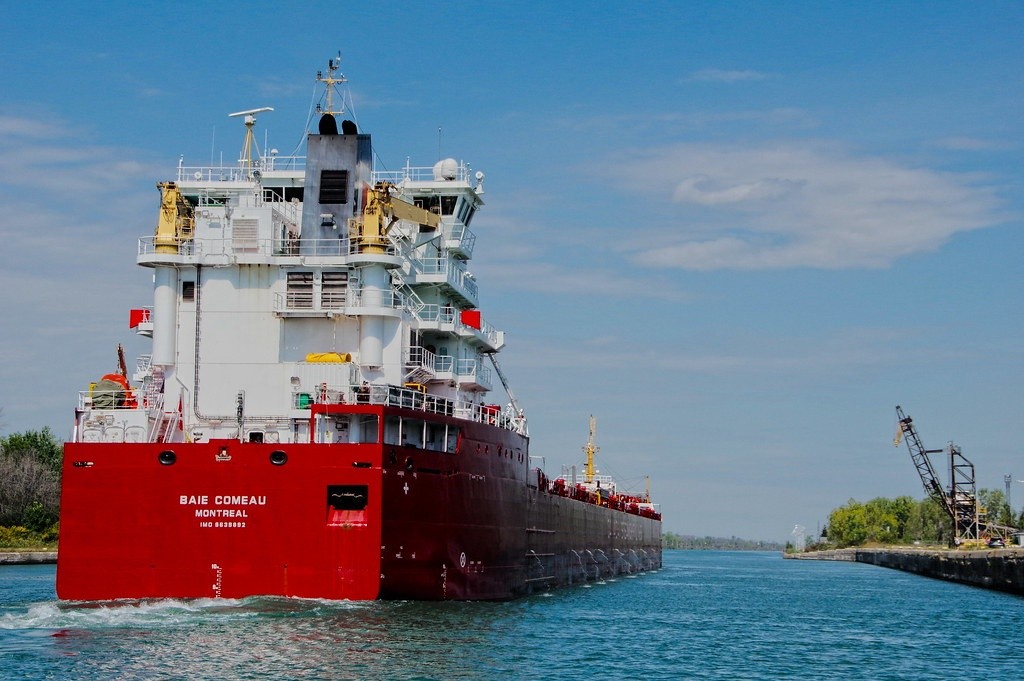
<box><xmin>989</xmin><ymin>537</ymin><xmax>1005</xmax><ymax>548</ymax></box>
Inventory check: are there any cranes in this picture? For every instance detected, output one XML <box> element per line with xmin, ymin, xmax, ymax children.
<box><xmin>895</xmin><ymin>405</ymin><xmax>980</xmax><ymax>551</ymax></box>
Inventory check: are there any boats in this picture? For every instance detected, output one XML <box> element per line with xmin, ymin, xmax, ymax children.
<box><xmin>53</xmin><ymin>47</ymin><xmax>664</xmax><ymax>622</ymax></box>
